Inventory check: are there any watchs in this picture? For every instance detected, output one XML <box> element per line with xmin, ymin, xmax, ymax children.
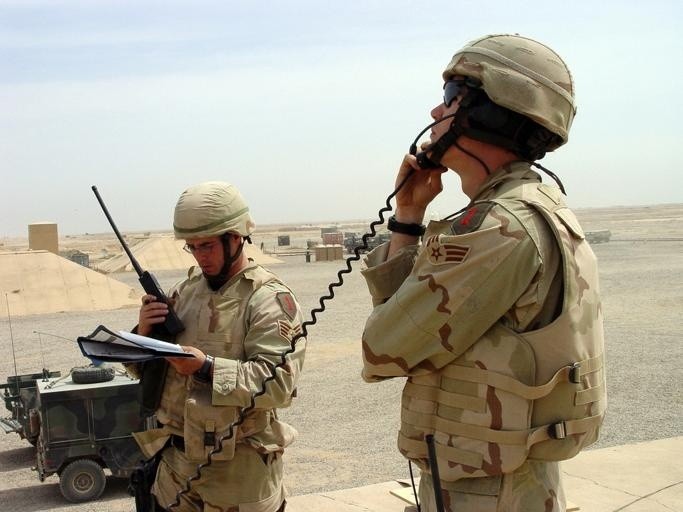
<box><xmin>194</xmin><ymin>353</ymin><xmax>213</xmax><ymax>384</ymax></box>
<box><xmin>387</xmin><ymin>214</ymin><xmax>426</xmax><ymax>236</ymax></box>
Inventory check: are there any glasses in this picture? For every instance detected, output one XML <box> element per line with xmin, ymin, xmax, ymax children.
<box><xmin>183</xmin><ymin>241</ymin><xmax>218</xmax><ymax>255</ymax></box>
<box><xmin>442</xmin><ymin>79</ymin><xmax>469</xmax><ymax>107</ymax></box>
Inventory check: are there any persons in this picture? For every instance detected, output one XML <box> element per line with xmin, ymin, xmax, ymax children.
<box><xmin>128</xmin><ymin>181</ymin><xmax>307</xmax><ymax>512</ymax></box>
<box><xmin>360</xmin><ymin>32</ymin><xmax>606</xmax><ymax>512</ymax></box>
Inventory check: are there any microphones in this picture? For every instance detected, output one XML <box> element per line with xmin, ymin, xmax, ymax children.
<box><xmin>408</xmin><ymin>113</ymin><xmax>455</xmax><ymax>154</ymax></box>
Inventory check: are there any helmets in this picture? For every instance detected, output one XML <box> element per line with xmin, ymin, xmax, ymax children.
<box><xmin>442</xmin><ymin>33</ymin><xmax>576</xmax><ymax>153</ymax></box>
<box><xmin>173</xmin><ymin>181</ymin><xmax>256</xmax><ymax>241</ymax></box>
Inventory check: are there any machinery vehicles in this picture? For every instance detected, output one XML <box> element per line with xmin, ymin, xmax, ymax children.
<box><xmin>26</xmin><ymin>366</ymin><xmax>154</xmax><ymax>503</ymax></box>
<box><xmin>344</xmin><ymin>233</ymin><xmax>391</xmax><ymax>256</ymax></box>
<box><xmin>0</xmin><ymin>368</ymin><xmax>121</xmax><ymax>450</ymax></box>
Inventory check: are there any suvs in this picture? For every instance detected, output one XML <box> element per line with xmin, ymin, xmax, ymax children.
<box><xmin>583</xmin><ymin>229</ymin><xmax>611</xmax><ymax>244</ymax></box>
